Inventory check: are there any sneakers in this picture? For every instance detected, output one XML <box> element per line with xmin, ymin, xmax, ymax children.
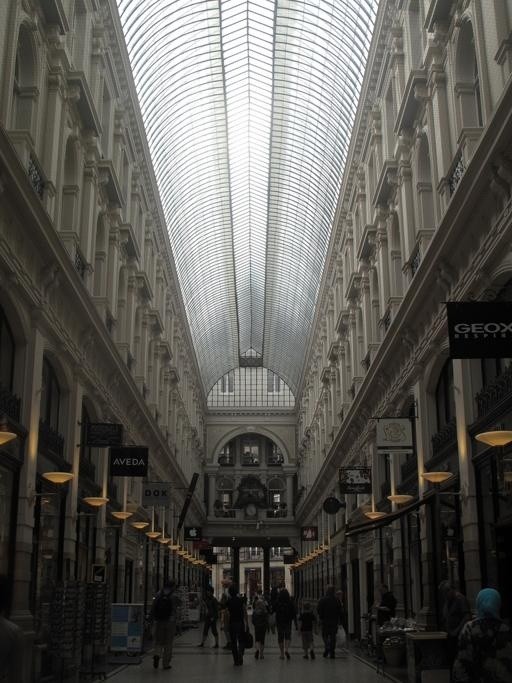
<box><xmin>162</xmin><ymin>664</ymin><xmax>172</xmax><ymax>670</ymax></box>
<box><xmin>212</xmin><ymin>643</ymin><xmax>219</xmax><ymax>648</ymax></box>
<box><xmin>152</xmin><ymin>654</ymin><xmax>160</xmax><ymax>670</ymax></box>
<box><xmin>303</xmin><ymin>650</ymin><xmax>315</xmax><ymax>660</ymax></box>
<box><xmin>197</xmin><ymin>642</ymin><xmax>204</xmax><ymax>647</ymax></box>
<box><xmin>325</xmin><ymin>649</ymin><xmax>335</xmax><ymax>659</ymax></box>
<box><xmin>279</xmin><ymin>652</ymin><xmax>290</xmax><ymax>660</ymax></box>
<box><xmin>255</xmin><ymin>650</ymin><xmax>264</xmax><ymax>660</ymax></box>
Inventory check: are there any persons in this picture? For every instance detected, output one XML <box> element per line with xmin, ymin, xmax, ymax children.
<box><xmin>198</xmin><ymin>579</ymin><xmax>348</xmax><ymax>666</ymax></box>
<box><xmin>438</xmin><ymin>582</ymin><xmax>471</xmax><ymax>634</ymax></box>
<box><xmin>455</xmin><ymin>589</ymin><xmax>511</xmax><ymax>682</ymax></box>
<box><xmin>374</xmin><ymin>584</ymin><xmax>397</xmax><ymax>663</ymax></box>
<box><xmin>148</xmin><ymin>578</ymin><xmax>179</xmax><ymax>669</ymax></box>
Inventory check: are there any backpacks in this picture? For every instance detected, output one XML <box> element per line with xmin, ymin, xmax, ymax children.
<box><xmin>154</xmin><ymin>590</ymin><xmax>173</xmax><ymax>623</ymax></box>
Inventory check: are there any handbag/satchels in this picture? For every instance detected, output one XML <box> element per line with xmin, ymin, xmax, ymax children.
<box><xmin>244</xmin><ymin>626</ymin><xmax>255</xmax><ymax>649</ymax></box>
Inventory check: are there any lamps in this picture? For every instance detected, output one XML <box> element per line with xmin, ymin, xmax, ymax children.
<box><xmin>0</xmin><ymin>417</ymin><xmax>213</xmax><ymax>572</ymax></box>
<box><xmin>386</xmin><ymin>494</ymin><xmax>426</xmax><ymax>521</ymax></box>
<box><xmin>420</xmin><ymin>471</ymin><xmax>468</xmax><ymax>500</ymax></box>
<box><xmin>287</xmin><ymin>543</ymin><xmax>332</xmax><ymax>573</ymax></box>
<box><xmin>474</xmin><ymin>429</ymin><xmax>512</xmax><ymax>501</ymax></box>
<box><xmin>364</xmin><ymin>510</ymin><xmax>399</xmax><ymax>529</ymax></box>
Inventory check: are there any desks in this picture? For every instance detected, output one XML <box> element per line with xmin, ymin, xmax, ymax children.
<box><xmin>405</xmin><ymin>630</ymin><xmax>457</xmax><ymax>683</ymax></box>
<box><xmin>376</xmin><ymin>627</ymin><xmax>425</xmax><ymax>677</ymax></box>
